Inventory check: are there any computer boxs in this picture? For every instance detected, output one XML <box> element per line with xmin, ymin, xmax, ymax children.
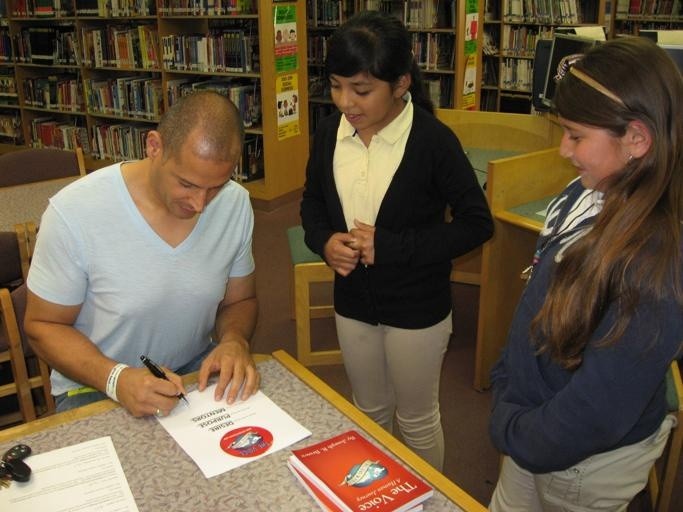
<box><xmin>533</xmin><ymin>39</ymin><xmax>552</xmax><ymax>112</ymax></box>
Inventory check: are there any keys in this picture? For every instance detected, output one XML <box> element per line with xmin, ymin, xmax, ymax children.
<box><xmin>0</xmin><ymin>444</ymin><xmax>31</xmax><ymax>489</ymax></box>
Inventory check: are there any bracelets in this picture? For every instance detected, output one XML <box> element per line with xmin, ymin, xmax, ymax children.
<box><xmin>107</xmin><ymin>363</ymin><xmax>128</xmax><ymax>402</ymax></box>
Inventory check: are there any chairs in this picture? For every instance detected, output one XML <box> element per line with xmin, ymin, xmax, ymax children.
<box><xmin>1</xmin><ymin>107</ymin><xmax>682</xmax><ymax>512</ymax></box>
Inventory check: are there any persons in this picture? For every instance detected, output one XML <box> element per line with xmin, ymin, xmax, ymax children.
<box><xmin>299</xmin><ymin>10</ymin><xmax>494</xmax><ymax>475</ymax></box>
<box><xmin>486</xmin><ymin>38</ymin><xmax>683</xmax><ymax>511</ymax></box>
<box><xmin>27</xmin><ymin>91</ymin><xmax>261</xmax><ymax>419</ymax></box>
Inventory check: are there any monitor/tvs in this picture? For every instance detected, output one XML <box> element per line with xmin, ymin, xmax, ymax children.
<box><xmin>541</xmin><ymin>33</ymin><xmax>600</xmax><ymax>107</ymax></box>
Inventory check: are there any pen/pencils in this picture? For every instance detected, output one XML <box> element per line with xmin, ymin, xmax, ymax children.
<box><xmin>140</xmin><ymin>355</ymin><xmax>191</xmax><ymax>405</ymax></box>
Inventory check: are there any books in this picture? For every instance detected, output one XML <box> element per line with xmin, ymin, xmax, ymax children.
<box><xmin>307</xmin><ymin>1</ymin><xmax>380</xmax><ymax>131</ymax></box>
<box><xmin>286</xmin><ymin>458</ymin><xmax>424</xmax><ymax>511</ymax></box>
<box><xmin>290</xmin><ymin>430</ymin><xmax>434</xmax><ymax>512</ymax></box>
<box><xmin>2</xmin><ymin>1</ymin><xmax>264</xmax><ymax>185</ymax></box>
<box><xmin>381</xmin><ymin>1</ymin><xmax>455</xmax><ymax>107</ymax></box>
<box><xmin>481</xmin><ymin>1</ymin><xmax>669</xmax><ymax>92</ymax></box>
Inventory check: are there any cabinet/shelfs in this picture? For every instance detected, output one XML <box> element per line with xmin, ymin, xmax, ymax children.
<box><xmin>484</xmin><ymin>0</ymin><xmax>606</xmax><ymax>114</ymax></box>
<box><xmin>605</xmin><ymin>0</ymin><xmax>683</xmax><ymax>41</ymax></box>
<box><xmin>307</xmin><ymin>0</ymin><xmax>485</xmax><ymax>141</ymax></box>
<box><xmin>1</xmin><ymin>1</ymin><xmax>311</xmax><ymax>213</ymax></box>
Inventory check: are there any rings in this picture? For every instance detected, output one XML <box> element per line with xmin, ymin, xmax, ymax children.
<box><xmin>156</xmin><ymin>409</ymin><xmax>161</xmax><ymax>417</ymax></box>
